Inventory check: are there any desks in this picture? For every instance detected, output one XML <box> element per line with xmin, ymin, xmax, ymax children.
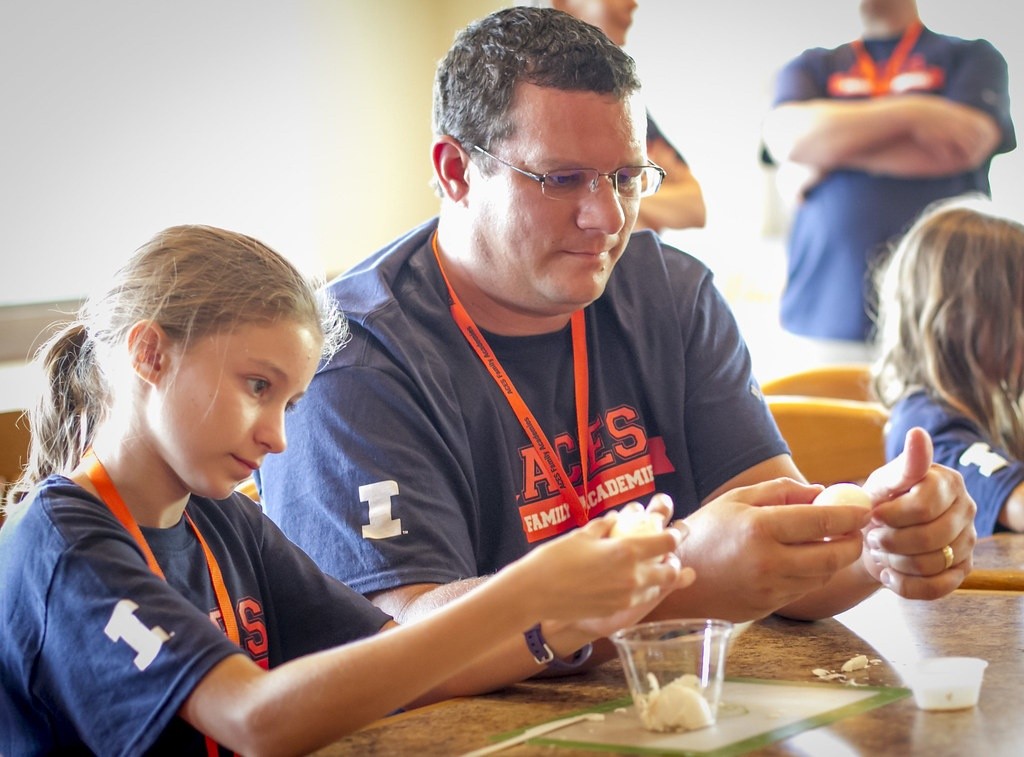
<box><xmin>310</xmin><ymin>590</ymin><xmax>1024</xmax><ymax>757</ymax></box>
<box><xmin>957</xmin><ymin>531</ymin><xmax>1024</xmax><ymax>591</ymax></box>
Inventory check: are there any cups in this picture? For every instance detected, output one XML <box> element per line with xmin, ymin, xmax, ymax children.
<box><xmin>607</xmin><ymin>618</ymin><xmax>733</xmax><ymax>731</ymax></box>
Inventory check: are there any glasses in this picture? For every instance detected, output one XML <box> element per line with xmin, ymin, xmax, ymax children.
<box><xmin>474</xmin><ymin>145</ymin><xmax>666</xmax><ymax>200</ymax></box>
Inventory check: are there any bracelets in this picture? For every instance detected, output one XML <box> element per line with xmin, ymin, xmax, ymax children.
<box><xmin>524</xmin><ymin>622</ymin><xmax>594</xmax><ymax>670</ymax></box>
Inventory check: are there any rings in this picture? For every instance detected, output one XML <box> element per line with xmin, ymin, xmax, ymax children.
<box><xmin>942</xmin><ymin>544</ymin><xmax>955</xmax><ymax>571</ymax></box>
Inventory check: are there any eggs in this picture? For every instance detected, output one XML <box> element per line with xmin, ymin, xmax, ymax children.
<box><xmin>607</xmin><ymin>511</ymin><xmax>665</xmax><ymax>563</ymax></box>
<box><xmin>812</xmin><ymin>483</ymin><xmax>871</xmax><ymax>511</ymax></box>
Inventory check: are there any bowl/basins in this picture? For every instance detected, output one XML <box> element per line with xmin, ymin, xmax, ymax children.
<box><xmin>904</xmin><ymin>657</ymin><xmax>989</xmax><ymax>710</ymax></box>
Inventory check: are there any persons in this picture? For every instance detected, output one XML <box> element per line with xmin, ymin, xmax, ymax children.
<box><xmin>754</xmin><ymin>0</ymin><xmax>1020</xmax><ymax>379</ymax></box>
<box><xmin>0</xmin><ymin>223</ymin><xmax>698</xmax><ymax>756</ymax></box>
<box><xmin>869</xmin><ymin>203</ymin><xmax>1024</xmax><ymax>538</ymax></box>
<box><xmin>525</xmin><ymin>0</ymin><xmax>706</xmax><ymax>238</ymax></box>
<box><xmin>253</xmin><ymin>6</ymin><xmax>981</xmax><ymax>678</ymax></box>
